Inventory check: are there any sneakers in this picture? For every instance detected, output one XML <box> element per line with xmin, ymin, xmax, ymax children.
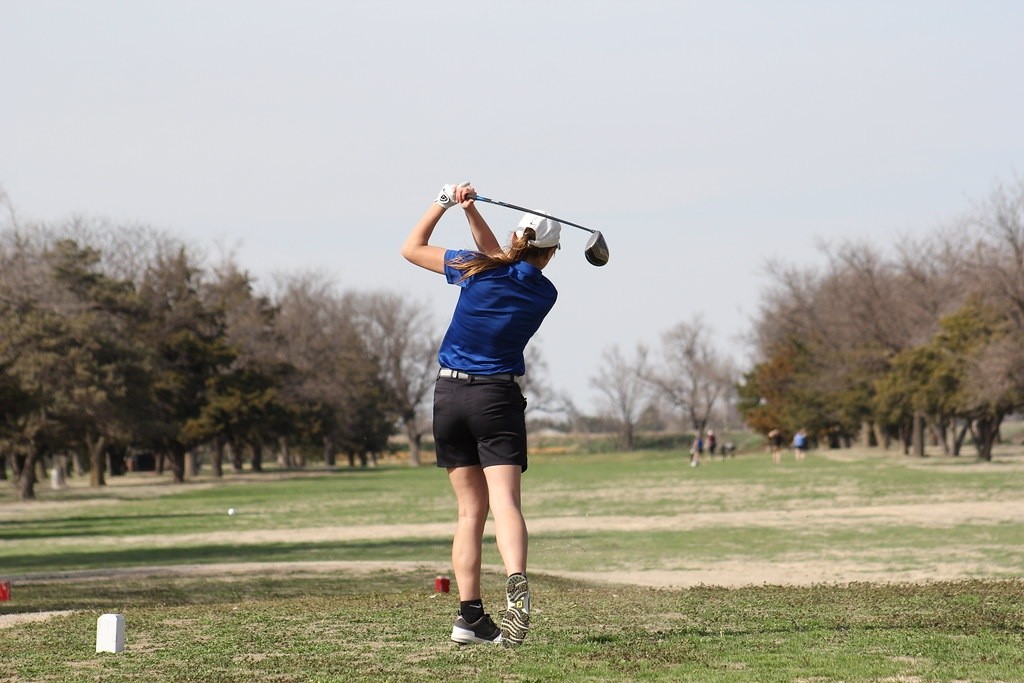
<box><xmin>451</xmin><ymin>613</ymin><xmax>503</xmax><ymax>644</ymax></box>
<box><xmin>500</xmin><ymin>575</ymin><xmax>531</xmax><ymax>650</ymax></box>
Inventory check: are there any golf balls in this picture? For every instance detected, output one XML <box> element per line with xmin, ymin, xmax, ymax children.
<box><xmin>228</xmin><ymin>507</ymin><xmax>235</xmax><ymax>516</ymax></box>
<box><xmin>760</xmin><ymin>397</ymin><xmax>766</xmax><ymax>405</ymax></box>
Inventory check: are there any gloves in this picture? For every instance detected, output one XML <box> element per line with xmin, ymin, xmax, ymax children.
<box><xmin>435</xmin><ymin>182</ymin><xmax>471</xmax><ymax>209</ymax></box>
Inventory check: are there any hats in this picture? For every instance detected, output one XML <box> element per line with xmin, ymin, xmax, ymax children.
<box><xmin>514</xmin><ymin>210</ymin><xmax>561</xmax><ymax>249</ymax></box>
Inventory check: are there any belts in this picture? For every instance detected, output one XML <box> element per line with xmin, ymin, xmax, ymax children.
<box><xmin>437</xmin><ymin>368</ymin><xmax>519</xmax><ymax>383</ymax></box>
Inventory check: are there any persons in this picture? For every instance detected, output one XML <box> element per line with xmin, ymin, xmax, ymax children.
<box><xmin>686</xmin><ymin>426</ymin><xmax>810</xmax><ymax>468</ymax></box>
<box><xmin>402</xmin><ymin>180</ymin><xmax>562</xmax><ymax>649</ymax></box>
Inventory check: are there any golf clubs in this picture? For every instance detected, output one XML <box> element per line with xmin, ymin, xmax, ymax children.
<box><xmin>464</xmin><ymin>194</ymin><xmax>609</xmax><ymax>267</ymax></box>
